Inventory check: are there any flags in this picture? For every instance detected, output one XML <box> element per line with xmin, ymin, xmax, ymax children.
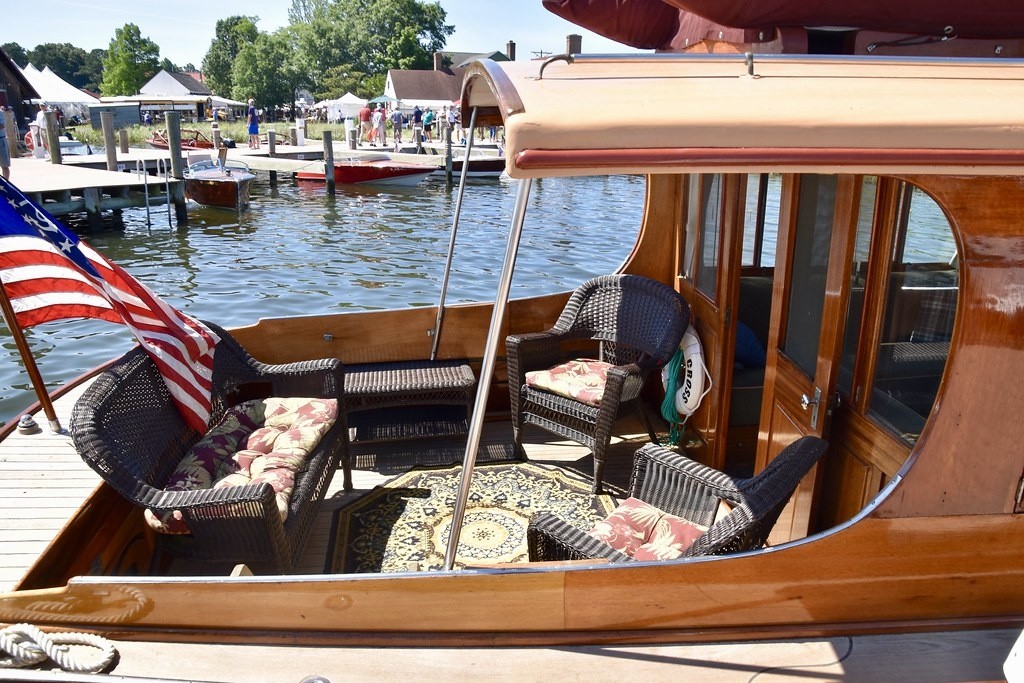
<box><xmin>0</xmin><ymin>176</ymin><xmax>222</xmax><ymax>435</ymax></box>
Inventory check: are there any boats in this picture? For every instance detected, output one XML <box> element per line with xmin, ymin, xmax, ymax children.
<box><xmin>428</xmin><ymin>157</ymin><xmax>506</xmax><ymax>183</ymax></box>
<box><xmin>0</xmin><ymin>35</ymin><xmax>1024</xmax><ymax>644</ymax></box>
<box><xmin>146</xmin><ymin>125</ymin><xmax>238</xmax><ymax>151</ymax></box>
<box><xmin>290</xmin><ymin>148</ymin><xmax>440</xmax><ymax>191</ymax></box>
<box><xmin>182</xmin><ymin>129</ymin><xmax>257</xmax><ymax>215</ymax></box>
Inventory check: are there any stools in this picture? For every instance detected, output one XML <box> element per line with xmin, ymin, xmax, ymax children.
<box><xmin>341</xmin><ymin>364</ymin><xmax>477</xmax><ymax>491</ymax></box>
<box><xmin>877</xmin><ymin>342</ymin><xmax>947</xmax><ymax>396</ymax></box>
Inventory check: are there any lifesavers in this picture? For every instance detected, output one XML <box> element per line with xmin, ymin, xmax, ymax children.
<box><xmin>187</xmin><ymin>137</ymin><xmax>197</xmax><ymax>147</ymax></box>
<box><xmin>660</xmin><ymin>324</ymin><xmax>706</xmax><ymax>415</ymax></box>
<box><xmin>24</xmin><ymin>131</ymin><xmax>37</xmax><ymax>151</ymax></box>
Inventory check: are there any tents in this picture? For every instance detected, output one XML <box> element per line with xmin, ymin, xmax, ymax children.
<box><xmin>333</xmin><ymin>92</ymin><xmax>367</xmax><ymax>119</ymax></box>
<box><xmin>11</xmin><ymin>59</ymin><xmax>100</xmax><ymax>126</ymax></box>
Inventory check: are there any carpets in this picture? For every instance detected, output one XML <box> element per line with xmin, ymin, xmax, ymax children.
<box><xmin>323</xmin><ymin>458</ymin><xmax>618</xmax><ymax>575</ymax></box>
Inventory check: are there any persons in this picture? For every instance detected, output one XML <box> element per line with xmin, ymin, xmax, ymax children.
<box><xmin>266</xmin><ymin>106</ymin><xmax>326</xmax><ymax>124</ymax></box>
<box><xmin>49</xmin><ymin>105</ymin><xmax>88</xmax><ymax>136</ymax></box>
<box><xmin>0</xmin><ymin>110</ymin><xmax>11</xmax><ymax>181</ymax></box>
<box><xmin>359</xmin><ymin>105</ymin><xmax>388</xmax><ymax>148</ymax></box>
<box><xmin>246</xmin><ymin>99</ymin><xmax>260</xmax><ymax>149</ymax></box>
<box><xmin>145</xmin><ymin>111</ymin><xmax>150</xmax><ymax>124</ymax></box>
<box><xmin>412</xmin><ymin>105</ymin><xmax>500</xmax><ymax>143</ymax></box>
<box><xmin>37</xmin><ymin>101</ymin><xmax>51</xmax><ymax>162</ymax></box>
<box><xmin>390</xmin><ymin>106</ymin><xmax>405</xmax><ymax>143</ymax></box>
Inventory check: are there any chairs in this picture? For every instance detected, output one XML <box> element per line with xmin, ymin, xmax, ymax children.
<box><xmin>506</xmin><ymin>274</ymin><xmax>691</xmax><ymax>496</ymax></box>
<box><xmin>526</xmin><ymin>436</ymin><xmax>831</xmax><ymax>563</ymax></box>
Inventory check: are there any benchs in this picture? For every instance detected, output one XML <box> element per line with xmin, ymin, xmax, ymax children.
<box><xmin>71</xmin><ymin>317</ymin><xmax>352</xmax><ymax>575</ymax></box>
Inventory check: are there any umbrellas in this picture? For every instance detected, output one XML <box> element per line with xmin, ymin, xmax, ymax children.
<box><xmin>370</xmin><ymin>95</ymin><xmax>399</xmax><ymax>109</ymax></box>
